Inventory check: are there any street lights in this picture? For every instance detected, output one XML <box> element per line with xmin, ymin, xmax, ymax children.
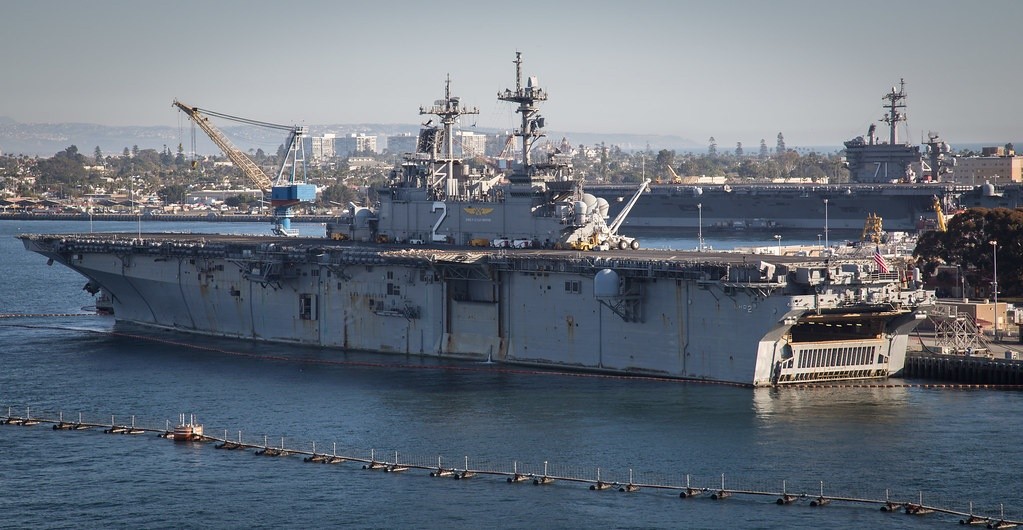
<box><xmin>990</xmin><ymin>240</ymin><xmax>1002</xmax><ymax>343</ymax></box>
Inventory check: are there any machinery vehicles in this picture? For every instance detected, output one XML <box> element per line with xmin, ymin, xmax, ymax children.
<box><xmin>666</xmin><ymin>164</ymin><xmax>682</xmax><ymax>184</ymax></box>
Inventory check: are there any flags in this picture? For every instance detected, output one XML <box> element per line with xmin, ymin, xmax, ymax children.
<box><xmin>874</xmin><ymin>245</ymin><xmax>890</xmax><ymax>275</ymax></box>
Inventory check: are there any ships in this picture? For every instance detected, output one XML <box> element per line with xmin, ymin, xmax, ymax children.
<box><xmin>578</xmin><ymin>77</ymin><xmax>960</xmax><ymax>242</ymax></box>
<box><xmin>11</xmin><ymin>49</ymin><xmax>938</xmax><ymax>395</ymax></box>
<box><xmin>671</xmin><ymin>188</ymin><xmax>955</xmax><ymax>266</ymax></box>
